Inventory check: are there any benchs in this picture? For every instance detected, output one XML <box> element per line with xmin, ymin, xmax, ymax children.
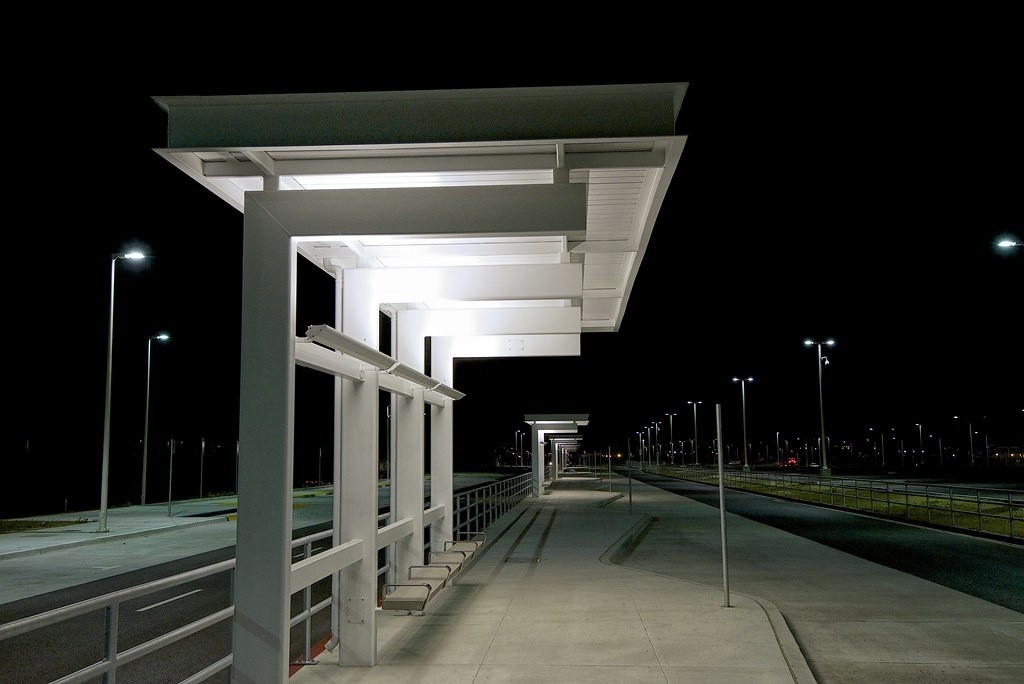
<box><xmin>381</xmin><ymin>532</ymin><xmax>487</xmax><ymax>616</ymax></box>
<box><xmin>542</xmin><ymin>478</ymin><xmax>553</xmax><ymax>487</ymax></box>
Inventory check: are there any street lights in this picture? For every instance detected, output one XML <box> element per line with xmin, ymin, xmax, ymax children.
<box><xmin>521</xmin><ymin>432</ymin><xmax>525</xmax><ymax>467</ymax></box>
<box><xmin>657</xmin><ymin>443</ymin><xmax>662</xmax><ymax>461</ymax></box>
<box><xmin>733</xmin><ymin>378</ymin><xmax>753</xmax><ymax>471</ymax></box>
<box><xmin>644</xmin><ymin>427</ymin><xmax>653</xmax><ymax>467</ymax></box>
<box><xmin>94</xmin><ymin>250</ymin><xmax>146</xmax><ymax>535</ymax></box>
<box><xmin>141</xmin><ymin>333</ymin><xmax>169</xmax><ymax>503</ymax></box>
<box><xmin>650</xmin><ymin>444</ymin><xmax>655</xmax><ymax>462</ymax></box>
<box><xmin>688</xmin><ymin>401</ymin><xmax>702</xmax><ymax>468</ymax></box>
<box><xmin>804</xmin><ymin>340</ymin><xmax>835</xmax><ymax>477</ymax></box>
<box><xmin>515</xmin><ymin>430</ymin><xmax>520</xmax><ymax>467</ymax></box>
<box><xmin>652</xmin><ymin>421</ymin><xmax>661</xmax><ymax>465</ymax></box>
<box><xmin>665</xmin><ymin>413</ymin><xmax>678</xmax><ymax>467</ymax></box>
<box><xmin>679</xmin><ymin>440</ymin><xmax>685</xmax><ymax>467</ymax></box>
<box><xmin>689</xmin><ymin>439</ymin><xmax>695</xmax><ymax>467</ymax></box>
<box><xmin>636</xmin><ymin>432</ymin><xmax>645</xmax><ymax>467</ymax></box>
